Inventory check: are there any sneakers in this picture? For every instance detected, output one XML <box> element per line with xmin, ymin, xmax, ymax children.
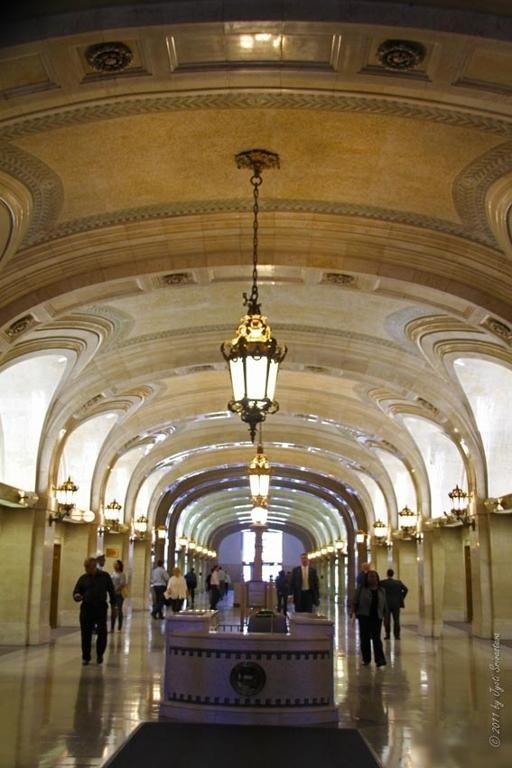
<box><xmin>109</xmin><ymin>629</ymin><xmax>121</xmax><ymax>633</ymax></box>
<box><xmin>82</xmin><ymin>656</ymin><xmax>104</xmax><ymax>665</ymax></box>
<box><xmin>362</xmin><ymin>661</ymin><xmax>386</xmax><ymax>666</ymax></box>
<box><xmin>151</xmin><ymin>611</ymin><xmax>166</xmax><ymax>619</ymax></box>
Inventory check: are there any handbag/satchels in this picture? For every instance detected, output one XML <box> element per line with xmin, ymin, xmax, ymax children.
<box><xmin>122</xmin><ymin>584</ymin><xmax>129</xmax><ymax>599</ymax></box>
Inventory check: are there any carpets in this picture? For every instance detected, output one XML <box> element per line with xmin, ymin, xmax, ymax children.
<box><xmin>100</xmin><ymin>722</ymin><xmax>384</xmax><ymax>768</ymax></box>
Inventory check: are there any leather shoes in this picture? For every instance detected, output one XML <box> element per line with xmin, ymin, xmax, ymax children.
<box><xmin>384</xmin><ymin>635</ymin><xmax>400</xmax><ymax>639</ymax></box>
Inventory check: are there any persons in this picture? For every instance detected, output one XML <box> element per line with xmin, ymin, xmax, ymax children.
<box><xmin>72</xmin><ymin>550</ymin><xmax>411</xmax><ymax>665</ymax></box>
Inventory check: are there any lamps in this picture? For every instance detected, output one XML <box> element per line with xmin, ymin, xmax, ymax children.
<box><xmin>220</xmin><ymin>148</ymin><xmax>288</xmax><ymax>444</ymax></box>
<box><xmin>321</xmin><ymin>536</ymin><xmax>347</xmax><ymax>562</ymax></box>
<box><xmin>100</xmin><ymin>498</ymin><xmax>122</xmax><ymax>536</ymax></box>
<box><xmin>131</xmin><ymin>514</ymin><xmax>148</xmax><ymax>545</ymax></box>
<box><xmin>246</xmin><ymin>422</ymin><xmax>272</xmax><ymax>528</ymax></box>
<box><xmin>448</xmin><ymin>484</ymin><xmax>475</xmax><ymax>531</ymax></box>
<box><xmin>48</xmin><ymin>476</ymin><xmax>77</xmax><ymax>526</ymax></box>
<box><xmin>151</xmin><ymin>521</ymin><xmax>195</xmax><ymax>555</ymax></box>
<box><xmin>356</xmin><ymin>504</ymin><xmax>421</xmax><ymax>550</ymax></box>
